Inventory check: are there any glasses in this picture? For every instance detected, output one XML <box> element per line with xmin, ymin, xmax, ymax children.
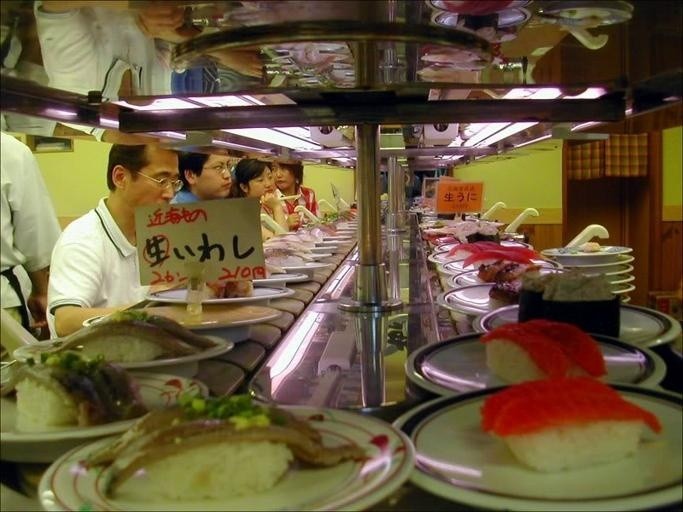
<box><xmin>128</xmin><ymin>169</ymin><xmax>184</xmax><ymax>193</ymax></box>
<box><xmin>196</xmin><ymin>159</ymin><xmax>235</xmax><ymax>173</ymax></box>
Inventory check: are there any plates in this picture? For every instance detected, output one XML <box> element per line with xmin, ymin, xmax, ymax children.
<box><xmin>471</xmin><ymin>304</ymin><xmax>683</xmax><ymax>350</ymax></box>
<box><xmin>83</xmin><ymin>305</ymin><xmax>283</xmax><ymax>346</ymax></box>
<box><xmin>0</xmin><ymin>372</ymin><xmax>209</xmax><ymax>461</ymax></box>
<box><xmin>405</xmin><ymin>331</ymin><xmax>667</xmax><ymax>399</ymax></box>
<box><xmin>437</xmin><ymin>284</ymin><xmax>520</xmax><ymax>318</ymax></box>
<box><xmin>37</xmin><ymin>404</ymin><xmax>415</xmax><ymax>510</ymax></box>
<box><xmin>14</xmin><ymin>333</ymin><xmax>236</xmax><ymax>378</ymax></box>
<box><xmin>391</xmin><ymin>380</ymin><xmax>683</xmax><ymax>512</ymax></box>
<box><xmin>147</xmin><ymin>285</ymin><xmax>295</xmax><ymax>307</ymax></box>
<box><xmin>409</xmin><ymin>205</ymin><xmax>564</xmax><ymax>288</ymax></box>
<box><xmin>541</xmin><ymin>246</ymin><xmax>635</xmax><ymax>306</ymax></box>
<box><xmin>254</xmin><ymin>214</ymin><xmax>357</xmax><ymax>289</ymax></box>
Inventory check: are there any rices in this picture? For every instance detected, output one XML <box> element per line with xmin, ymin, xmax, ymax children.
<box><xmin>12</xmin><ymin>379</ymin><xmax>78</xmax><ymax>433</ymax></box>
<box><xmin>505</xmin><ymin>418</ymin><xmax>650</xmax><ymax>473</ymax></box>
<box><xmin>112</xmin><ymin>436</ymin><xmax>296</xmax><ymax>503</ymax></box>
<box><xmin>455</xmin><ymin>249</ymin><xmax>473</xmax><ymax>260</ymax></box>
<box><xmin>266</xmin><ymin>254</ymin><xmax>306</xmax><ymax>268</ymax></box>
<box><xmin>80</xmin><ymin>332</ymin><xmax>161</xmax><ymax>364</ymax></box>
<box><xmin>485</xmin><ymin>338</ymin><xmax>543</xmax><ymax>384</ymax></box>
<box><xmin>473</xmin><ymin>259</ymin><xmax>497</xmax><ymax>270</ymax></box>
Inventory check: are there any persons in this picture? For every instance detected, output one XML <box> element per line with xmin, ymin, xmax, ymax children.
<box><xmin>230</xmin><ymin>157</ymin><xmax>289</xmax><ymax>242</ymax></box>
<box><xmin>48</xmin><ymin>142</ymin><xmax>181</xmax><ymax>338</ymax></box>
<box><xmin>33</xmin><ymin>0</ymin><xmax>273</xmax><ymax>148</ymax></box>
<box><xmin>1</xmin><ymin>130</ymin><xmax>61</xmax><ymax>339</ymax></box>
<box><xmin>264</xmin><ymin>158</ymin><xmax>320</xmax><ymax>230</ymax></box>
<box><xmin>167</xmin><ymin>148</ymin><xmax>232</xmax><ymax>204</ymax></box>
